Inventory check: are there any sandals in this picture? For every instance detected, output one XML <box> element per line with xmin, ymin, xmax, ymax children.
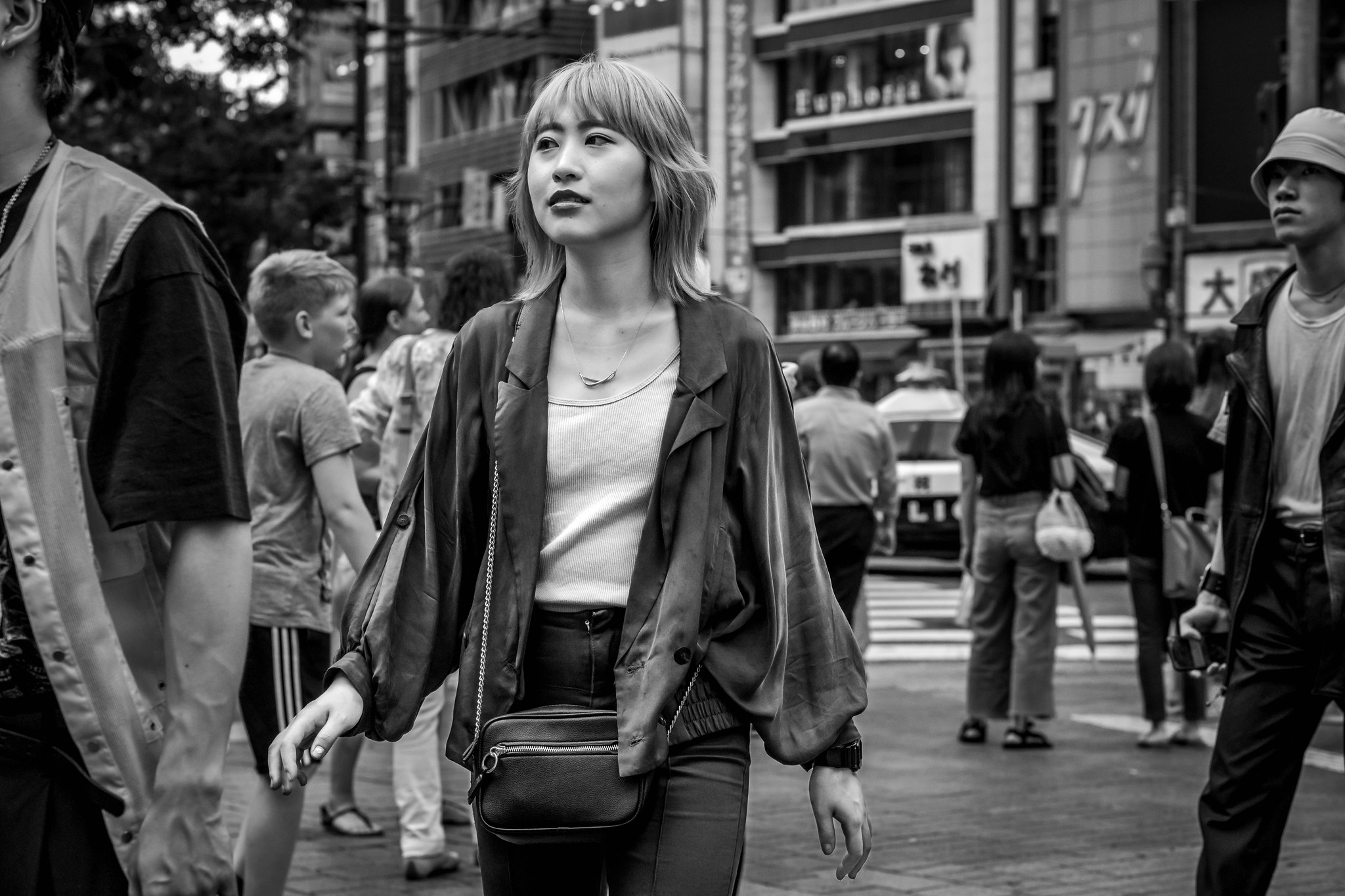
<box><xmin>319</xmin><ymin>800</ymin><xmax>385</xmax><ymax>837</ymax></box>
<box><xmin>959</xmin><ymin>718</ymin><xmax>987</xmax><ymax>743</ymax></box>
<box><xmin>1002</xmin><ymin>727</ymin><xmax>1054</xmax><ymax>750</ymax></box>
<box><xmin>442</xmin><ymin>799</ymin><xmax>472</xmax><ymax>827</ymax></box>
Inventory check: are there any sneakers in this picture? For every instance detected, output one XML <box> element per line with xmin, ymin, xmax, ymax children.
<box><xmin>405</xmin><ymin>852</ymin><xmax>460</xmax><ymax>881</ymax></box>
<box><xmin>474</xmin><ymin>849</ymin><xmax>479</xmax><ymax>867</ymax></box>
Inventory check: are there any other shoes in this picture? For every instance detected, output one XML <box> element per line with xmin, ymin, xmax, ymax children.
<box><xmin>1137</xmin><ymin>729</ymin><xmax>1214</xmax><ymax>749</ymax></box>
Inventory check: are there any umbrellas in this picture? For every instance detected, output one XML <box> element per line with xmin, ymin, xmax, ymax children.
<box><xmin>1068</xmin><ymin>556</ymin><xmax>1097</xmax><ymax>660</ymax></box>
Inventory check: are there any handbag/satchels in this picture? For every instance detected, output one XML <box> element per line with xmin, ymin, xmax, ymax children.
<box><xmin>468</xmin><ymin>706</ymin><xmax>650</xmax><ymax>844</ymax></box>
<box><xmin>1162</xmin><ymin>518</ymin><xmax>1220</xmax><ymax>597</ymax></box>
<box><xmin>1035</xmin><ymin>488</ymin><xmax>1094</xmax><ymax>561</ymax></box>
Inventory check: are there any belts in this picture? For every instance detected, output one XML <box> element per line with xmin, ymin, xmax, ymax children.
<box><xmin>1265</xmin><ymin>520</ymin><xmax>1323</xmax><ymax>546</ymax></box>
<box><xmin>0</xmin><ymin>731</ymin><xmax>126</xmax><ymax>817</ymax></box>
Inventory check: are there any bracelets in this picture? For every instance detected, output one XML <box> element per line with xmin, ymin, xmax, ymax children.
<box><xmin>959</xmin><ymin>537</ymin><xmax>972</xmax><ymax>549</ymax></box>
<box><xmin>815</xmin><ymin>740</ymin><xmax>864</xmax><ymax>772</ymax></box>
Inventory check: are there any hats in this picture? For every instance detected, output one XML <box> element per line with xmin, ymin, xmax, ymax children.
<box><xmin>1250</xmin><ymin>107</ymin><xmax>1345</xmax><ymax>209</ymax></box>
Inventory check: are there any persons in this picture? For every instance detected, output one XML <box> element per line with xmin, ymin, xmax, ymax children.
<box><xmin>270</xmin><ymin>56</ymin><xmax>878</xmax><ymax>896</ymax></box>
<box><xmin>0</xmin><ymin>1</ymin><xmax>254</xmax><ymax>896</ymax></box>
<box><xmin>1178</xmin><ymin>106</ymin><xmax>1345</xmax><ymax>896</ymax></box>
<box><xmin>953</xmin><ymin>330</ymin><xmax>1077</xmax><ymax>749</ymax></box>
<box><xmin>232</xmin><ymin>248</ymin><xmax>900</xmax><ymax>896</ymax></box>
<box><xmin>1103</xmin><ymin>324</ymin><xmax>1238</xmax><ymax>751</ymax></box>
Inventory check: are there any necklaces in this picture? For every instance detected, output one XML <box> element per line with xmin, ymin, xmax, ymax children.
<box><xmin>0</xmin><ymin>133</ymin><xmax>57</xmax><ymax>242</ymax></box>
<box><xmin>558</xmin><ymin>288</ymin><xmax>659</xmax><ymax>388</ymax></box>
<box><xmin>1297</xmin><ymin>279</ymin><xmax>1345</xmax><ymax>304</ymax></box>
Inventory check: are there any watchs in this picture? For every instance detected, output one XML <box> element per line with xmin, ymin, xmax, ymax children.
<box><xmin>1196</xmin><ymin>561</ymin><xmax>1232</xmax><ymax>605</ymax></box>
